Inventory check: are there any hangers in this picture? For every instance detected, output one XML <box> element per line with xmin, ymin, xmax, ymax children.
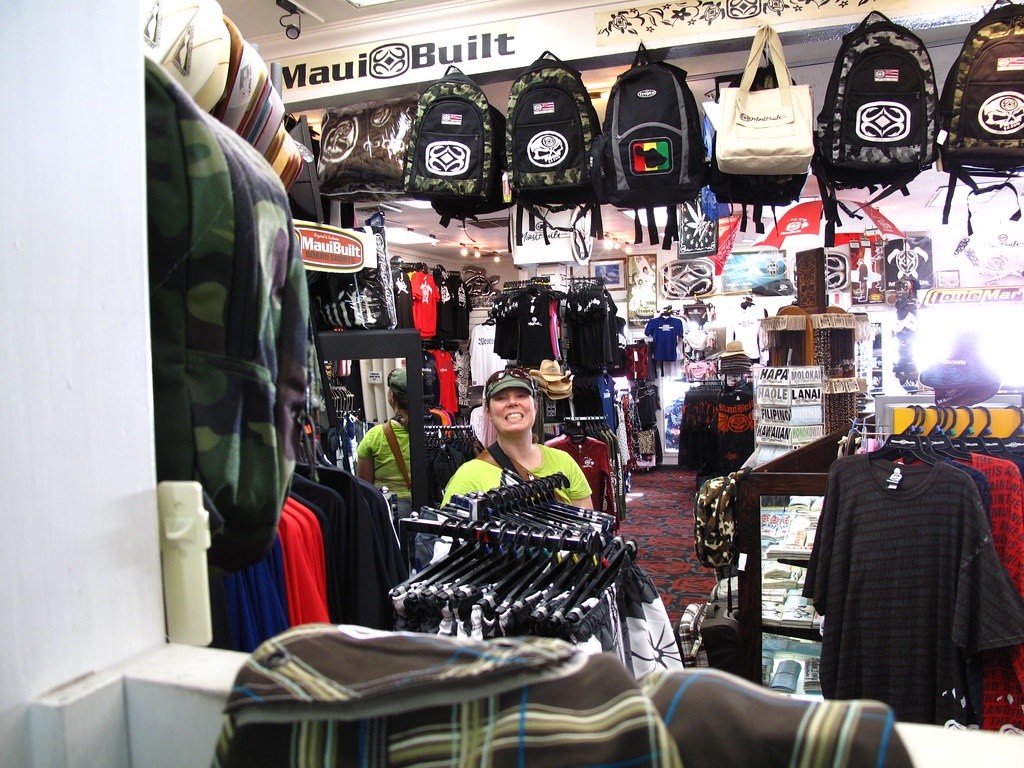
<box><xmin>842</xmin><ymin>405</ymin><xmax>1024</xmax><ymax>466</ymax></box>
<box><xmin>330</xmin><ymin>386</ymin><xmax>361</xmax><ymax>419</ymax></box>
<box><xmin>417</xmin><ymin>424</ymin><xmax>473</xmax><ymax>442</ymax></box>
<box><xmin>661</xmin><ymin>305</ymin><xmax>675</xmax><ymax>319</ymax></box>
<box><xmin>686</xmin><ymin>377</ymin><xmax>724</xmax><ymax>396</ymax></box>
<box><xmin>561</xmin><ymin>410</ymin><xmax>610</xmax><ymax>435</ymax></box>
<box><xmin>389</xmin><ymin>472</ymin><xmax>640</xmax><ymax>625</ymax></box>
<box><xmin>500</xmin><ymin>275</ymin><xmax>605</xmax><ymax>295</ymax></box>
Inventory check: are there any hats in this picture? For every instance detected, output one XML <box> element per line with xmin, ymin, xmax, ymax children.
<box><xmin>385</xmin><ymin>367</ymin><xmax>408</xmax><ymax>398</ymax></box>
<box><xmin>529</xmin><ymin>359</ymin><xmax>573</xmax><ymax>401</ymax></box>
<box><xmin>920</xmin><ymin>330</ymin><xmax>1000</xmax><ymax>407</ymax></box>
<box><xmin>719</xmin><ymin>341</ymin><xmax>752</xmax><ymax>375</ymax></box>
<box><xmin>485</xmin><ymin>370</ymin><xmax>534</xmax><ymax>402</ymax></box>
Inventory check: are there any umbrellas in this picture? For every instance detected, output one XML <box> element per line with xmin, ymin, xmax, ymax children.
<box><xmin>753</xmin><ymin>199</ymin><xmax>907</xmax><ymax>306</ymax></box>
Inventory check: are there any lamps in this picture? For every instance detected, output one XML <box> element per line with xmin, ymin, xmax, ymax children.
<box><xmin>603</xmin><ymin>232</ymin><xmax>633</xmax><ymax>256</ymax></box>
<box><xmin>473</xmin><ymin>245</ymin><xmax>481</xmax><ymax>258</ymax></box>
<box><xmin>272</xmin><ymin>0</ymin><xmax>309</xmax><ymax>43</ymax></box>
<box><xmin>459</xmin><ymin>243</ymin><xmax>469</xmax><ymax>257</ymax></box>
<box><xmin>492</xmin><ymin>251</ymin><xmax>502</xmax><ymax>264</ymax></box>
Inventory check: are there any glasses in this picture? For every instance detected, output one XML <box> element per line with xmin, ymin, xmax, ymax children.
<box><xmin>388</xmin><ymin>369</ymin><xmax>396</xmax><ymax>387</ymax></box>
<box><xmin>485</xmin><ymin>370</ymin><xmax>531</xmax><ymax>396</ymax></box>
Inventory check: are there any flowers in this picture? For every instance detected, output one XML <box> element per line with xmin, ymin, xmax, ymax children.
<box><xmin>391</xmin><ymin>256</ymin><xmax>463</xmax><ymax>281</ymax></box>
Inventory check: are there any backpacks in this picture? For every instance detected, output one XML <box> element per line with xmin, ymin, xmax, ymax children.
<box><xmin>601</xmin><ymin>43</ymin><xmax>706</xmax><ymax>250</ymax></box>
<box><xmin>146</xmin><ymin>55</ymin><xmax>312</xmax><ymax>564</ymax></box>
<box><xmin>312</xmin><ymin>226</ymin><xmax>398</xmax><ymax>330</ymax></box>
<box><xmin>401</xmin><ymin>64</ymin><xmax>515</xmax><ymax>242</ymax></box>
<box><xmin>505</xmin><ymin>50</ymin><xmax>606</xmax><ymax>239</ymax></box>
<box><xmin>692</xmin><ymin>466</ymin><xmax>753</xmax><ymax>567</ymax></box>
<box><xmin>814</xmin><ymin>10</ymin><xmax>939</xmax><ymax>247</ymax></box>
<box><xmin>937</xmin><ymin>0</ymin><xmax>1024</xmax><ymax>177</ymax></box>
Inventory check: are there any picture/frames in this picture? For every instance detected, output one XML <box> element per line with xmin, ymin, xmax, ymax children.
<box><xmin>721</xmin><ymin>248</ymin><xmax>789</xmax><ymax>294</ymax></box>
<box><xmin>625</xmin><ymin>253</ymin><xmax>658</xmax><ymax>331</ymax></box>
<box><xmin>588</xmin><ymin>257</ymin><xmax>628</xmax><ymax>292</ymax></box>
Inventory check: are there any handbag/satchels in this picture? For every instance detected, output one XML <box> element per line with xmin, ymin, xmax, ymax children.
<box><xmin>317</xmin><ymin>92</ymin><xmax>420</xmax><ymax>203</ymax></box>
<box><xmin>702</xmin><ymin>25</ymin><xmax>816</xmax><ymax>208</ymax></box>
<box><xmin>209</xmin><ymin>622</ymin><xmax>916</xmax><ymax>768</ymax></box>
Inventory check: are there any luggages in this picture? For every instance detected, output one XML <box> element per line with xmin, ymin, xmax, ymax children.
<box><xmin>673</xmin><ymin>565</ymin><xmax>742</xmax><ymax>679</ymax></box>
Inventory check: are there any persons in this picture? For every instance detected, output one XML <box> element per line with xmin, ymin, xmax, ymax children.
<box><xmin>443</xmin><ymin>368</ymin><xmax>593</xmax><ymax>511</ymax></box>
<box><xmin>358</xmin><ymin>368</ymin><xmax>411</xmax><ymax>519</ymax></box>
